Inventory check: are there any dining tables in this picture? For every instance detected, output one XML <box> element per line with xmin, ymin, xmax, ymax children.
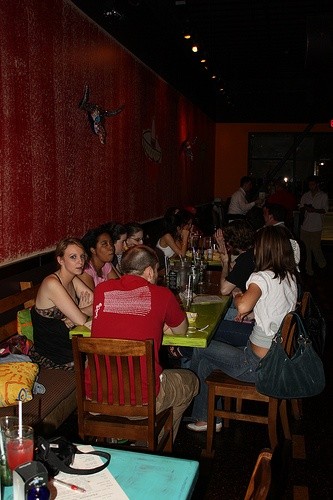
<box><xmin>68</xmin><ymin>258</ymin><xmax>234</xmax><ymax>369</ymax></box>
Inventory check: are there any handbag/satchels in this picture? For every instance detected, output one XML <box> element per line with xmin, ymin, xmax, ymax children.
<box><xmin>255</xmin><ymin>311</ymin><xmax>325</xmax><ymax>399</ymax></box>
<box><xmin>32</xmin><ymin>436</ymin><xmax>111</xmax><ymax>480</ymax></box>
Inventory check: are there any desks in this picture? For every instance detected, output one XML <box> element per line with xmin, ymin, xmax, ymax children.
<box><xmin>0</xmin><ymin>432</ymin><xmax>200</xmax><ymax>500</ymax></box>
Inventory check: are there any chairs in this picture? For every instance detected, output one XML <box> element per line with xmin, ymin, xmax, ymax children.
<box><xmin>199</xmin><ymin>303</ymin><xmax>303</xmax><ymax>462</ymax></box>
<box><xmin>71</xmin><ymin>335</ymin><xmax>174</xmax><ymax>458</ymax></box>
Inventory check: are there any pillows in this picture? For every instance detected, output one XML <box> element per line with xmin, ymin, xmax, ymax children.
<box><xmin>0</xmin><ymin>363</ymin><xmax>40</xmax><ymax>409</ymax></box>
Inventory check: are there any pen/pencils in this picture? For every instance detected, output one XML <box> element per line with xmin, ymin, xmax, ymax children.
<box><xmin>50</xmin><ymin>478</ymin><xmax>86</xmax><ymax>493</ymax></box>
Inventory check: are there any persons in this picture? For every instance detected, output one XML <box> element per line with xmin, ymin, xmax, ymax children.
<box><xmin>255</xmin><ymin>202</ymin><xmax>274</xmax><ymax>231</ymax></box>
<box><xmin>81</xmin><ymin>245</ymin><xmax>200</xmax><ymax>452</ymax></box>
<box><xmin>167</xmin><ymin>299</ymin><xmax>256</xmax><ymax>372</ymax></box>
<box><xmin>151</xmin><ymin>207</ymin><xmax>195</xmax><ymax>270</ymax></box>
<box><xmin>183</xmin><ymin>224</ymin><xmax>298</xmax><ymax>434</ymax></box>
<box><xmin>77</xmin><ymin>227</ymin><xmax>120</xmax><ymax>294</ymax></box>
<box><xmin>269</xmin><ymin>180</ymin><xmax>295</xmax><ymax>209</ymax></box>
<box><xmin>214</xmin><ymin>219</ymin><xmax>265</xmax><ymax>324</ymax></box>
<box><xmin>267</xmin><ymin>205</ymin><xmax>293</xmax><ymax>238</ymax></box>
<box><xmin>296</xmin><ymin>174</ymin><xmax>329</xmax><ymax>276</ymax></box>
<box><xmin>102</xmin><ymin>221</ymin><xmax>128</xmax><ymax>267</ymax></box>
<box><xmin>227</xmin><ymin>175</ymin><xmax>264</xmax><ymax>225</ymax></box>
<box><xmin>121</xmin><ymin>221</ymin><xmax>146</xmax><ymax>253</ymax></box>
<box><xmin>29</xmin><ymin>237</ymin><xmax>95</xmax><ymax>365</ymax></box>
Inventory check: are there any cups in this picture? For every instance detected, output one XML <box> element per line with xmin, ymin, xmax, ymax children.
<box><xmin>164</xmin><ymin>234</ymin><xmax>218</xmax><ymax>313</ymax></box>
<box><xmin>4</xmin><ymin>425</ymin><xmax>35</xmax><ymax>471</ymax></box>
<box><xmin>0</xmin><ymin>416</ymin><xmax>21</xmax><ymax>488</ymax></box>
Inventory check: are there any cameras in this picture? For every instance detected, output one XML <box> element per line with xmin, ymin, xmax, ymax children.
<box><xmin>12</xmin><ymin>462</ymin><xmax>50</xmax><ymax>500</ymax></box>
<box><xmin>34</xmin><ymin>435</ymin><xmax>78</xmax><ymax>480</ymax></box>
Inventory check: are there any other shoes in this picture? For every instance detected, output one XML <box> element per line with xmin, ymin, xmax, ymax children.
<box><xmin>187</xmin><ymin>421</ymin><xmax>223</xmax><ymax>433</ymax></box>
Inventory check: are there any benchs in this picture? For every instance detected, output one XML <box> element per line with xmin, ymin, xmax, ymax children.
<box><xmin>0</xmin><ymin>281</ymin><xmax>78</xmax><ymax>441</ymax></box>
